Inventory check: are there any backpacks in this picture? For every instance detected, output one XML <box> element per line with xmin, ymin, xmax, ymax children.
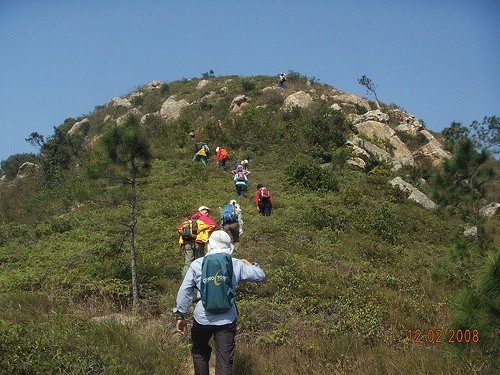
<box><xmin>260</xmin><ymin>187</ymin><xmax>269</xmax><ymax>201</ymax></box>
<box><xmin>282</xmin><ymin>78</ymin><xmax>286</xmax><ymax>83</ymax></box>
<box><xmin>237</xmin><ymin>172</ymin><xmax>245</xmax><ymax>181</ymax></box>
<box><xmin>221</xmin><ymin>149</ymin><xmax>230</xmax><ymax>159</ymax></box>
<box><xmin>224</xmin><ymin>204</ymin><xmax>236</xmax><ymax>223</ymax></box>
<box><xmin>241</xmin><ymin>160</ymin><xmax>246</xmax><ymax>165</ymax></box>
<box><xmin>200</xmin><ymin>253</ymin><xmax>234</xmax><ymax>314</ymax></box>
<box><xmin>182</xmin><ymin>213</ymin><xmax>203</xmax><ymax>241</ymax></box>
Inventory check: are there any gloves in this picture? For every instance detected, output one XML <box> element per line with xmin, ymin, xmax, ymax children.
<box><xmin>239</xmin><ymin>229</ymin><xmax>243</xmax><ymax>237</ymax></box>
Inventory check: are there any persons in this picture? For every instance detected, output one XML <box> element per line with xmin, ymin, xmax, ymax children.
<box><xmin>176</xmin><ymin>230</ymin><xmax>266</xmax><ymax>375</ymax></box>
<box><xmin>255</xmin><ymin>184</ymin><xmax>273</xmax><ymax>217</ymax></box>
<box><xmin>216</xmin><ymin>145</ymin><xmax>227</xmax><ymax>172</ymax></box>
<box><xmin>178</xmin><ymin>206</ymin><xmax>222</xmax><ymax>288</ymax></box>
<box><xmin>192</xmin><ymin>142</ymin><xmax>210</xmax><ymax>167</ymax></box>
<box><xmin>231</xmin><ymin>159</ymin><xmax>251</xmax><ymax>196</ymax></box>
<box><xmin>278</xmin><ymin>72</ymin><xmax>287</xmax><ymax>88</ymax></box>
<box><xmin>221</xmin><ymin>199</ymin><xmax>243</xmax><ymax>255</ymax></box>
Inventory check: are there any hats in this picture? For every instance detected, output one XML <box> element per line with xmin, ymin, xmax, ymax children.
<box><xmin>205</xmin><ymin>230</ymin><xmax>231</xmax><ymax>249</ymax></box>
<box><xmin>237</xmin><ymin>165</ymin><xmax>243</xmax><ymax>169</ymax></box>
<box><xmin>198</xmin><ymin>206</ymin><xmax>213</xmax><ymax>213</ymax></box>
<box><xmin>230</xmin><ymin>200</ymin><xmax>236</xmax><ymax>204</ymax></box>
<box><xmin>215</xmin><ymin>146</ymin><xmax>220</xmax><ymax>152</ymax></box>
